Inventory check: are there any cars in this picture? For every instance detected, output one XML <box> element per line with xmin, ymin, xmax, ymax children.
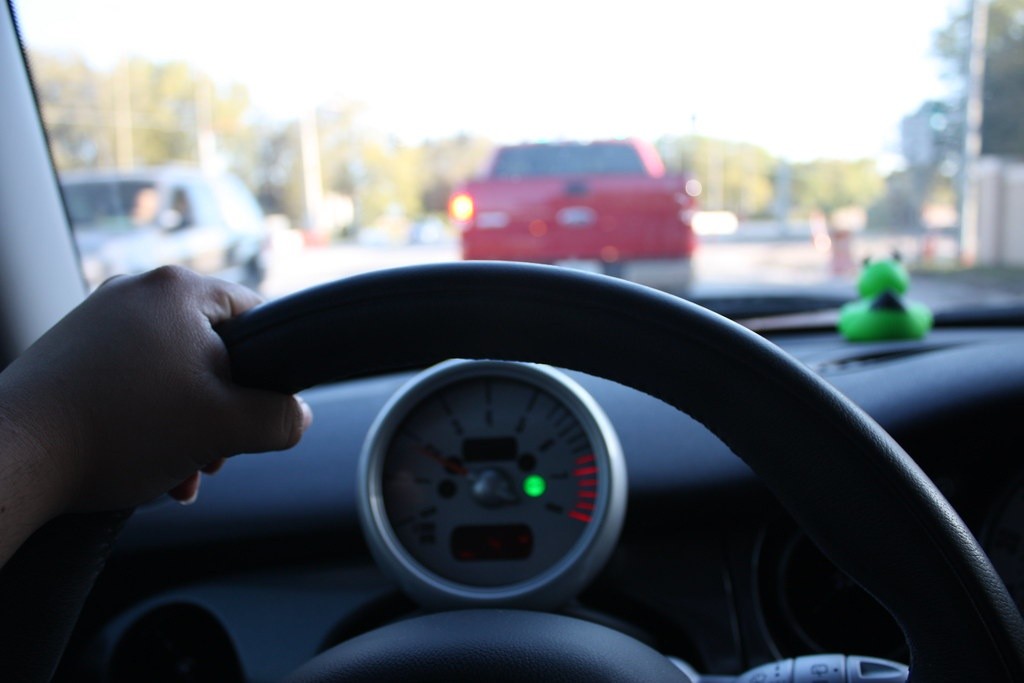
<box><xmin>59</xmin><ymin>167</ymin><xmax>270</xmax><ymax>289</ymax></box>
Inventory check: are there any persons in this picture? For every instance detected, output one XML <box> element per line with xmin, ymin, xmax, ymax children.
<box><xmin>0</xmin><ymin>264</ymin><xmax>313</xmax><ymax>573</ymax></box>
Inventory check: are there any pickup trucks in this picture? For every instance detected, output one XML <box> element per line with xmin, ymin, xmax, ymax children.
<box><xmin>453</xmin><ymin>136</ymin><xmax>698</xmax><ymax>294</ymax></box>
